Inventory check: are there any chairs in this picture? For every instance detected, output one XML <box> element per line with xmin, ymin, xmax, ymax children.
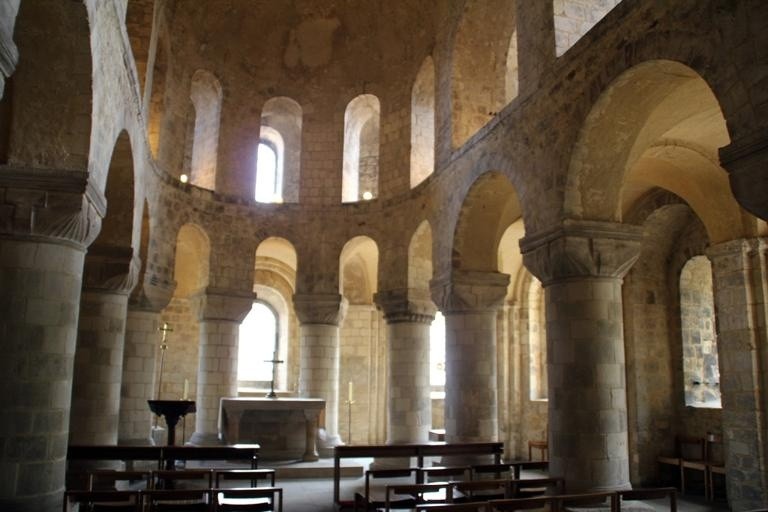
<box><xmin>528</xmin><ymin>430</ymin><xmax>549</xmax><ymax>470</ymax></box>
<box><xmin>658</xmin><ymin>436</ymin><xmax>727</xmax><ymax>504</ymax></box>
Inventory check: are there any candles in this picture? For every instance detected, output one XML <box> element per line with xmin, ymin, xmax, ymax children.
<box><xmin>348</xmin><ymin>382</ymin><xmax>352</xmax><ymax>402</ymax></box>
<box><xmin>183</xmin><ymin>379</ymin><xmax>188</xmax><ymax>399</ymax></box>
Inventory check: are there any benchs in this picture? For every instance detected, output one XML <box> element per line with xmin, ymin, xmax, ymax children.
<box><xmin>379</xmin><ymin>477</ymin><xmax>566</xmax><ymax>512</ymax></box>
<box><xmin>91</xmin><ymin>469</ymin><xmax>277</xmax><ymax>489</ymax></box>
<box><xmin>354</xmin><ymin>463</ymin><xmax>521</xmax><ymax>511</ymax></box>
<box><xmin>67</xmin><ymin>443</ymin><xmax>260</xmax><ymax>503</ymax></box>
<box><xmin>78</xmin><ymin>486</ymin><xmax>283</xmax><ymax>512</ymax></box>
<box><xmin>415</xmin><ymin>486</ymin><xmax>679</xmax><ymax>511</ymax></box>
<box><xmin>332</xmin><ymin>441</ymin><xmax>504</xmax><ymax>510</ymax></box>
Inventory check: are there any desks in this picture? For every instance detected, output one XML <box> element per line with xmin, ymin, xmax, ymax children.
<box><xmin>148</xmin><ymin>400</ymin><xmax>197</xmax><ymax>467</ymax></box>
<box><xmin>222</xmin><ymin>397</ymin><xmax>326</xmax><ymax>462</ymax></box>
